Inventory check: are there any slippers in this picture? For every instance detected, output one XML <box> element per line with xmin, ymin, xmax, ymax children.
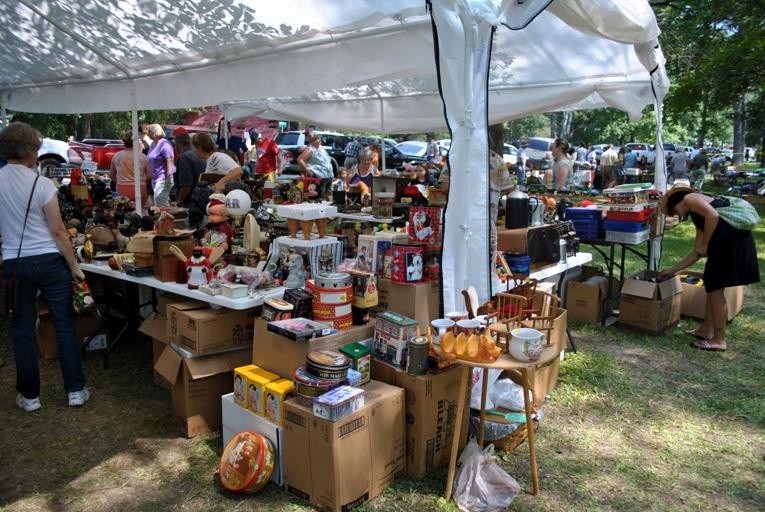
<box><xmin>689</xmin><ymin>338</ymin><xmax>728</xmax><ymax>352</ymax></box>
<box><xmin>685</xmin><ymin>328</ymin><xmax>706</xmax><ymax>339</ymax></box>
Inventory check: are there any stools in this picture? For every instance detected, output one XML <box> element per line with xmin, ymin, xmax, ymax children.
<box><xmin>277</xmin><ymin>202</ymin><xmax>338</xmax><ymax>240</ymax></box>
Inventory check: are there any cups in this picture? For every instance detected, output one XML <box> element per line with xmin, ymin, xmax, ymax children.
<box><xmin>255</xmin><ymin>262</ymin><xmax>277</xmax><ymax>275</ymax></box>
<box><xmin>506</xmin><ymin>186</ymin><xmax>538</xmax><ymax>230</ymax></box>
<box><xmin>428</xmin><ymin>310</ymin><xmax>485</xmax><ymax>344</ymax></box>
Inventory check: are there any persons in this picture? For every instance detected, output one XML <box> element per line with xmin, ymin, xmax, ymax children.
<box><xmin>110</xmin><ymin>121</ymin><xmax>437</xmax><ymax>229</ymax></box>
<box><xmin>657</xmin><ymin>186</ymin><xmax>760</xmax><ymax>350</ymax></box>
<box><xmin>0</xmin><ymin>123</ymin><xmax>90</xmax><ymax>411</ymax></box>
<box><xmin>516</xmin><ymin>136</ymin><xmax>749</xmax><ymax>195</ymax></box>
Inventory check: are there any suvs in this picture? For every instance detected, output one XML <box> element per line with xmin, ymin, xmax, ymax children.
<box><xmin>31</xmin><ymin>135</ymin><xmax>73</xmax><ymax>188</ymax></box>
<box><xmin>270</xmin><ymin>125</ymin><xmax>349</xmax><ymax>174</ymax></box>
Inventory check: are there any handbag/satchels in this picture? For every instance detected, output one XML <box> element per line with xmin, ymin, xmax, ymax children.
<box><xmin>707</xmin><ymin>193</ymin><xmax>761</xmax><ymax>232</ymax></box>
<box><xmin>0</xmin><ymin>276</ymin><xmax>21</xmax><ymax>323</ymax></box>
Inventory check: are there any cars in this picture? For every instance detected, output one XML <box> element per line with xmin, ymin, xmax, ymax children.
<box><xmin>696</xmin><ymin>143</ymin><xmax>758</xmax><ymax>163</ymax></box>
<box><xmin>428</xmin><ymin>137</ymin><xmax>453</xmax><ymax>149</ymax></box>
<box><xmin>650</xmin><ymin>141</ymin><xmax>679</xmax><ymax>162</ymax></box>
<box><xmin>380</xmin><ymin>137</ymin><xmax>450</xmax><ymax>169</ymax></box>
<box><xmin>586</xmin><ymin>144</ymin><xmax>611</xmax><ymax>164</ymax></box>
<box><xmin>496</xmin><ymin>141</ymin><xmax>532</xmax><ymax>174</ymax></box>
<box><xmin>507</xmin><ymin>137</ymin><xmax>579</xmax><ymax>172</ymax></box>
<box><xmin>677</xmin><ymin>145</ymin><xmax>697</xmax><ymax>160</ymax></box>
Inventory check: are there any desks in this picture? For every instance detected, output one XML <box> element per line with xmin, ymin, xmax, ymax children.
<box><xmin>262</xmin><ymin>202</ymin><xmax>403</xmax><ymax>224</ymax></box>
<box><xmin>78</xmin><ymin>262</ymin><xmax>304</xmax><ymax>370</ymax></box>
<box><xmin>443</xmin><ymin>342</ymin><xmax>559</xmax><ymax>501</ymax></box>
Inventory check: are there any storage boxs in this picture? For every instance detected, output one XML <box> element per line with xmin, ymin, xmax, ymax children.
<box><xmin>625</xmin><ymin>168</ymin><xmax>639</xmax><ymax>176</ymax></box>
<box><xmin>33</xmin><ymin>307</ymin><xmax>58</xmax><ymax>360</ymax></box>
<box><xmin>675</xmin><ymin>268</ymin><xmax>744</xmax><ymax>323</ymax></box>
<box><xmin>564</xmin><ymin>208</ymin><xmax>653</xmax><ymax>245</ymax></box>
<box><xmin>568</xmin><ymin>272</ymin><xmax>616</xmax><ymax>324</ymax></box>
<box><xmin>154</xmin><ymin>240</ymin><xmax>198</xmax><ymax>284</ymax></box>
<box><xmin>503</xmin><ymin>306</ymin><xmax>568</xmax><ymax>407</ymax></box>
<box><xmin>272</xmin><ymin>233</ymin><xmax>343</xmax><ymax>279</ymax></box>
<box><xmin>618</xmin><ymin>269</ymin><xmax>683</xmax><ymax>335</ymax></box>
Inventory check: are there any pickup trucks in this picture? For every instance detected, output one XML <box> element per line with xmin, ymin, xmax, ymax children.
<box><xmin>70</xmin><ymin>121</ymin><xmax>245</xmax><ymax>198</ymax></box>
<box><xmin>624</xmin><ymin>142</ymin><xmax>656</xmax><ymax>165</ymax></box>
<box><xmin>283</xmin><ymin>132</ymin><xmax>427</xmax><ymax>179</ymax></box>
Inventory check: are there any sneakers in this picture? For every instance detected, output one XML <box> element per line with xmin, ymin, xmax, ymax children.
<box><xmin>67</xmin><ymin>384</ymin><xmax>90</xmax><ymax>407</ymax></box>
<box><xmin>14</xmin><ymin>392</ymin><xmax>41</xmax><ymax>413</ymax></box>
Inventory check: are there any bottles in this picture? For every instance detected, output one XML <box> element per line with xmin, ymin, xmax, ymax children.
<box><xmin>370</xmin><ymin>191</ymin><xmax>395</xmax><ymax>220</ymax></box>
<box><xmin>528</xmin><ymin>199</ymin><xmax>546</xmax><ymax>226</ymax></box>
<box><xmin>509</xmin><ymin>327</ymin><xmax>544</xmax><ymax>362</ymax></box>
<box><xmin>407</xmin><ymin>333</ymin><xmax>430</xmax><ymax>376</ymax></box>
<box><xmin>84</xmin><ymin>234</ymin><xmax>95</xmax><ymax>262</ymax></box>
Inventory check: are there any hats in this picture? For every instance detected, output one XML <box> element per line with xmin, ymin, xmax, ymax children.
<box><xmin>661</xmin><ymin>181</ymin><xmax>694</xmax><ymax>218</ymax></box>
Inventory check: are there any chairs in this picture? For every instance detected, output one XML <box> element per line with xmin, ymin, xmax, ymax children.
<box><xmin>460</xmin><ymin>275</ymin><xmax>560</xmax><ymax>353</ymax></box>
